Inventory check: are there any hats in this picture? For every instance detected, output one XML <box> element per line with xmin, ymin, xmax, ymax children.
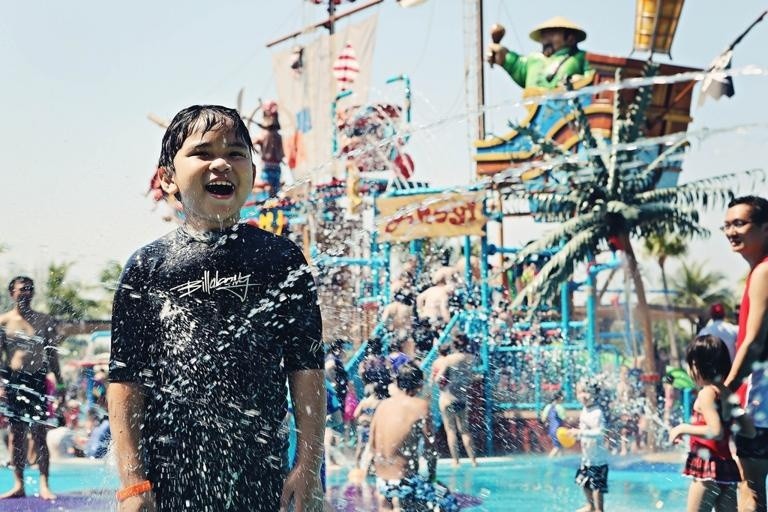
<box><xmin>390</xmin><ymin>355</ymin><xmax>411</xmax><ymax>370</ymax></box>
<box><xmin>528</xmin><ymin>16</ymin><xmax>586</xmax><ymax>42</ymax></box>
<box><xmin>710</xmin><ymin>304</ymin><xmax>726</xmax><ymax>315</ymax></box>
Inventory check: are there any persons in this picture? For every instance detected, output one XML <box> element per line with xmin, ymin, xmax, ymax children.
<box><xmin>670</xmin><ymin>333</ymin><xmax>743</xmax><ymax>512</ymax></box>
<box><xmin>486</xmin><ymin>19</ymin><xmax>594</xmax><ymax>113</ymax></box>
<box><xmin>65</xmin><ymin>373</ymin><xmax>112</xmax><ymax>458</ymax></box>
<box><xmin>386</xmin><ymin>342</ymin><xmax>408</xmax><ymax>379</ymax></box>
<box><xmin>102</xmin><ymin>105</ymin><xmax>328</xmax><ymax>512</ymax></box>
<box><xmin>429</xmin><ymin>343</ymin><xmax>452</xmax><ymax>383</ymax></box>
<box><xmin>719</xmin><ymin>195</ymin><xmax>768</xmax><ymax>512</ymax></box>
<box><xmin>539</xmin><ymin>390</ymin><xmax>571</xmax><ymax>461</ymax></box>
<box><xmin>437</xmin><ymin>331</ymin><xmax>485</xmax><ymax>470</ymax></box>
<box><xmin>347</xmin><ymin>357</ymin><xmax>462</xmax><ymax>512</ymax></box>
<box><xmin>1</xmin><ymin>274</ymin><xmax>65</xmax><ymax>502</ymax></box>
<box><xmin>379</xmin><ymin>242</ymin><xmax>501</xmax><ymax>346</ymax></box>
<box><xmin>700</xmin><ymin>303</ymin><xmax>739</xmax><ymax>367</ymax></box>
<box><xmin>325</xmin><ymin>339</ymin><xmax>348</xmax><ymax>471</ymax></box>
<box><xmin>353</xmin><ymin>383</ymin><xmax>382</xmax><ymax>469</ymax></box>
<box><xmin>358</xmin><ymin>339</ymin><xmax>391</xmax><ymax>388</ymax></box>
<box><xmin>571</xmin><ymin>378</ymin><xmax>609</xmax><ymax>512</ymax></box>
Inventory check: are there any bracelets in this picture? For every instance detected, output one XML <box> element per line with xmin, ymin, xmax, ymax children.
<box><xmin>112</xmin><ymin>480</ymin><xmax>153</xmax><ymax>501</ymax></box>
